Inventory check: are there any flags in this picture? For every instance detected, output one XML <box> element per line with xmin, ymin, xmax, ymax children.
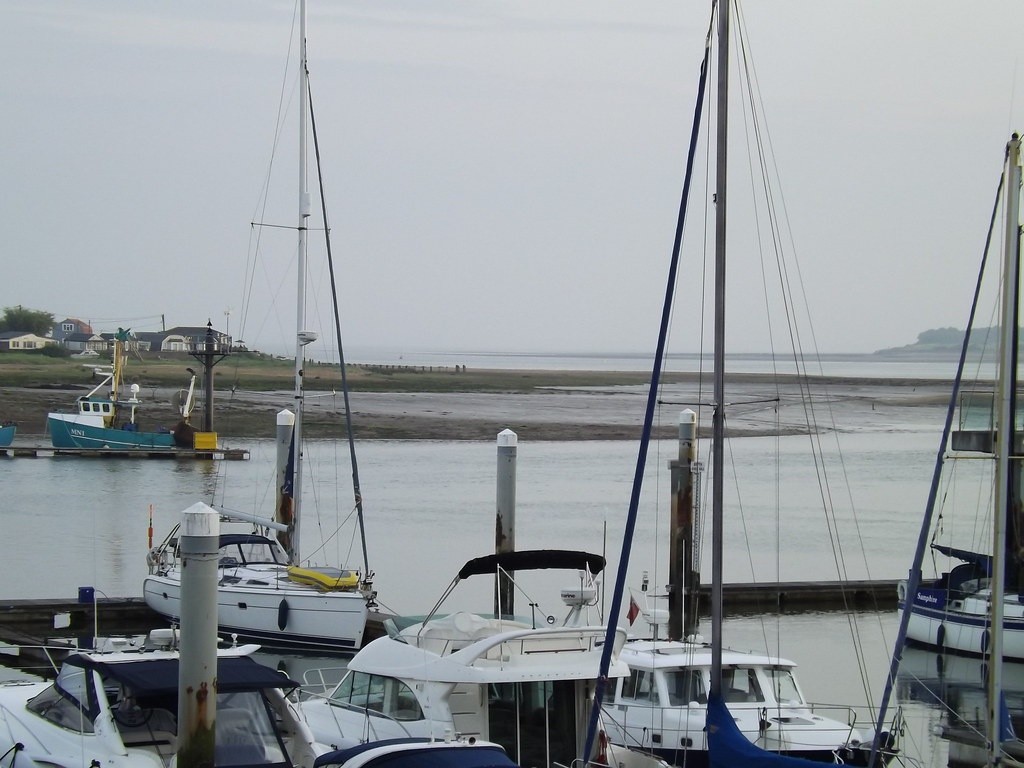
<box><xmin>626</xmin><ymin>595</ymin><xmax>639</xmax><ymax>626</ymax></box>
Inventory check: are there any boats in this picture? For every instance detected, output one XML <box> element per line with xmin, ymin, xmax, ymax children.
<box><xmin>0</xmin><ymin>624</ymin><xmax>521</xmax><ymax>768</ymax></box>
<box><xmin>594</xmin><ymin>608</ymin><xmax>901</xmax><ymax>768</ymax></box>
<box><xmin>0</xmin><ymin>421</ymin><xmax>17</xmax><ymax>455</ymax></box>
<box><xmin>47</xmin><ymin>340</ymin><xmax>197</xmax><ymax>461</ymax></box>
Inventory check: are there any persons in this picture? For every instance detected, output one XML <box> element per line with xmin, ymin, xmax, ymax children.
<box><xmin>722</xmin><ymin>669</ymin><xmax>747</xmax><ymax>702</ymax></box>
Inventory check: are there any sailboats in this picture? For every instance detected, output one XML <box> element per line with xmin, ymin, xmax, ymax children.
<box><xmin>294</xmin><ymin>0</ymin><xmax>1024</xmax><ymax>768</ymax></box>
<box><xmin>142</xmin><ymin>2</ymin><xmax>378</xmax><ymax>657</ymax></box>
<box><xmin>894</xmin><ymin>645</ymin><xmax>1024</xmax><ymax>724</ymax></box>
<box><xmin>896</xmin><ymin>219</ymin><xmax>1024</xmax><ymax>664</ymax></box>
